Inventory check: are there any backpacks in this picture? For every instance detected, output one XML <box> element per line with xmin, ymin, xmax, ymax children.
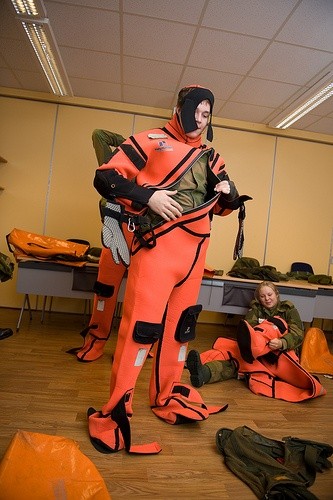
<box><xmin>216</xmin><ymin>424</ymin><xmax>333</xmax><ymax>500</ymax></box>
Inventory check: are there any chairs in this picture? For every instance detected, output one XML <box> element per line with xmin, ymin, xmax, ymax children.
<box><xmin>232</xmin><ymin>257</ymin><xmax>260</xmax><ymax>268</ymax></box>
<box><xmin>290</xmin><ymin>262</ymin><xmax>315</xmax><ymax>275</ymax></box>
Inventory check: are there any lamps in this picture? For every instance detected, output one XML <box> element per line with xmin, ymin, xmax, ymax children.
<box><xmin>263</xmin><ymin>60</ymin><xmax>333</xmax><ymax>130</ymax></box>
<box><xmin>20</xmin><ymin>18</ymin><xmax>74</xmax><ymax>97</ymax></box>
<box><xmin>12</xmin><ymin>0</ymin><xmax>48</xmax><ymax>18</ymax></box>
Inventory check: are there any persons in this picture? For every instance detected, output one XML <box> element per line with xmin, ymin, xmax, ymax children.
<box><xmin>87</xmin><ymin>84</ymin><xmax>241</xmax><ymax>453</ymax></box>
<box><xmin>186</xmin><ymin>281</ymin><xmax>304</xmax><ymax>387</ymax></box>
<box><xmin>77</xmin><ymin>129</ymin><xmax>159</xmax><ymax>362</ymax></box>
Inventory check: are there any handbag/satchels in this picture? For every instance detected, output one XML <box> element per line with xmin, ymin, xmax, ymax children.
<box><xmin>230</xmin><ymin>257</ymin><xmax>290</xmax><ymax>283</ymax></box>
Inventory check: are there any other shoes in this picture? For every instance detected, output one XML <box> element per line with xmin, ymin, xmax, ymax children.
<box><xmin>186</xmin><ymin>350</ymin><xmax>205</xmax><ymax>388</ymax></box>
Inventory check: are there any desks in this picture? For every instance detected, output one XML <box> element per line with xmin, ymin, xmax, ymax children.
<box><xmin>15</xmin><ymin>258</ymin><xmax>333</xmax><ymax>336</ymax></box>
